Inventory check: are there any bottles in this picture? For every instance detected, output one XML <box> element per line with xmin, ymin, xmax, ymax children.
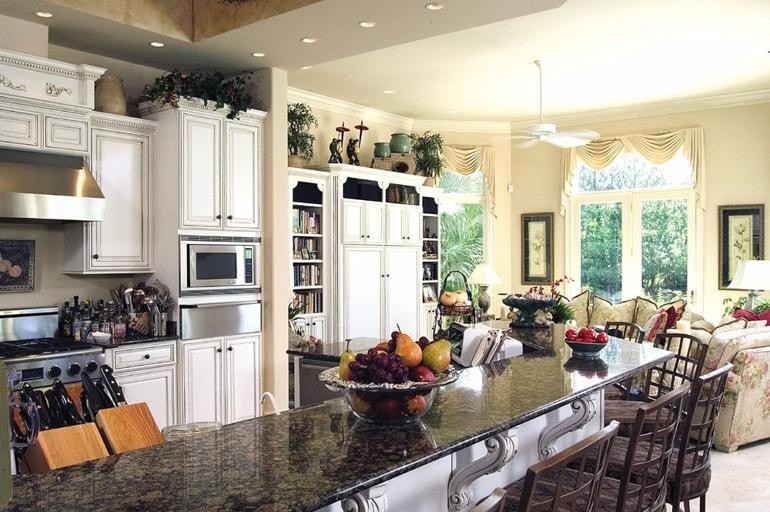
<box><xmin>114</xmin><ymin>305</ymin><xmax>126</xmax><ymax>339</ymax></box>
<box><xmin>72</xmin><ymin>296</ymin><xmax>82</xmax><ymax>338</ymax></box>
<box><xmin>63</xmin><ymin>302</ymin><xmax>72</xmax><ymax>336</ymax></box>
<box><xmin>89</xmin><ymin>298</ymin><xmax>95</xmax><ymax>318</ymax></box>
<box><xmin>82</xmin><ymin>301</ymin><xmax>90</xmax><ymax>320</ymax></box>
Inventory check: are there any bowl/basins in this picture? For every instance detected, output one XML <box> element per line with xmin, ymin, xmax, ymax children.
<box><xmin>92</xmin><ymin>333</ymin><xmax>111</xmax><ymax>343</ymax></box>
<box><xmin>563</xmin><ymin>340</ymin><xmax>605</xmax><ymax>359</ymax></box>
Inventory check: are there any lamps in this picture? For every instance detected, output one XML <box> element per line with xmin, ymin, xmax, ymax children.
<box><xmin>725</xmin><ymin>258</ymin><xmax>770</xmax><ymax>312</ymax></box>
<box><xmin>467</xmin><ymin>261</ymin><xmax>500</xmax><ymax>313</ymax></box>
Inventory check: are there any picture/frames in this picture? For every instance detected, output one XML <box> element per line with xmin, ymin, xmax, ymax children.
<box><xmin>0</xmin><ymin>240</ymin><xmax>36</xmax><ymax>293</ymax></box>
<box><xmin>716</xmin><ymin>203</ymin><xmax>766</xmax><ymax>292</ymax></box>
<box><xmin>519</xmin><ymin>212</ymin><xmax>556</xmax><ymax>286</ymax></box>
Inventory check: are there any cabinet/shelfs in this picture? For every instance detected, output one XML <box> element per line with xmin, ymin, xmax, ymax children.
<box><xmin>288</xmin><ymin>167</ymin><xmax>333</xmax><ymax>346</ymax></box>
<box><xmin>420</xmin><ymin>185</ymin><xmax>440</xmax><ymax>343</ymax></box>
<box><xmin>327</xmin><ymin>166</ymin><xmax>421</xmax><ymax>357</ymax></box>
<box><xmin>61</xmin><ymin>115</ymin><xmax>155</xmax><ymax>276</ymax></box>
<box><xmin>0</xmin><ymin>93</ymin><xmax>93</xmax><ymax>158</ymax></box>
<box><xmin>176</xmin><ymin>332</ymin><xmax>262</xmax><ymax>428</ymax></box>
<box><xmin>131</xmin><ymin>97</ymin><xmax>270</xmax><ymax>230</ymax></box>
<box><xmin>103</xmin><ymin>339</ymin><xmax>176</xmax><ymax>430</ymax></box>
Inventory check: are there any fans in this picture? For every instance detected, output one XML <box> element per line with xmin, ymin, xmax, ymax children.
<box><xmin>489</xmin><ymin>60</ymin><xmax>600</xmax><ymax>148</ymax></box>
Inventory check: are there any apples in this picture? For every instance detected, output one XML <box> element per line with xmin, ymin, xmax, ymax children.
<box><xmin>410</xmin><ymin>366</ymin><xmax>435</xmax><ymax>382</ymax></box>
<box><xmin>377</xmin><ymin>399</ymin><xmax>401</xmax><ymax>419</ymax></box>
<box><xmin>356</xmin><ymin>389</ymin><xmax>383</xmax><ymax>401</ymax></box>
<box><xmin>565</xmin><ymin>327</ymin><xmax>608</xmax><ymax>343</ymax></box>
<box><xmin>399</xmin><ymin>391</ymin><xmax>426</xmax><ymax>416</ymax></box>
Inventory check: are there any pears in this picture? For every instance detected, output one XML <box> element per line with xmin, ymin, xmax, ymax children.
<box><xmin>351</xmin><ymin>393</ymin><xmax>370</xmax><ymax>414</ymax></box>
<box><xmin>339</xmin><ymin>351</ymin><xmax>357</xmax><ymax>381</ymax></box>
<box><xmin>395</xmin><ymin>334</ymin><xmax>423</xmax><ymax>369</ymax></box>
<box><xmin>423</xmin><ymin>339</ymin><xmax>452</xmax><ymax>373</ymax></box>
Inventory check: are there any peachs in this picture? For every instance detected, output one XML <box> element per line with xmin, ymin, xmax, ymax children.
<box><xmin>441</xmin><ymin>291</ymin><xmax>472</xmax><ymax>312</ymax></box>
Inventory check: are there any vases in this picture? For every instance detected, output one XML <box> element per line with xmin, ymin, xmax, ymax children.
<box><xmin>390</xmin><ymin>134</ymin><xmax>410</xmax><ymax>153</ymax></box>
<box><xmin>372</xmin><ymin>142</ymin><xmax>391</xmax><ymax>158</ymax></box>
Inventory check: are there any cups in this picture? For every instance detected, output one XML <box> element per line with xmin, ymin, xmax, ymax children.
<box><xmin>72</xmin><ymin>322</ymin><xmax>82</xmax><ymax>335</ymax></box>
<box><xmin>92</xmin><ymin>324</ymin><xmax>99</xmax><ymax>332</ymax></box>
<box><xmin>101</xmin><ymin>323</ymin><xmax>111</xmax><ymax>332</ymax></box>
<box><xmin>83</xmin><ymin>321</ymin><xmax>91</xmax><ymax>335</ymax></box>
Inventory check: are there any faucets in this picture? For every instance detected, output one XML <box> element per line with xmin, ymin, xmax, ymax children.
<box><xmin>259</xmin><ymin>392</ymin><xmax>280</xmax><ymax>415</ymax></box>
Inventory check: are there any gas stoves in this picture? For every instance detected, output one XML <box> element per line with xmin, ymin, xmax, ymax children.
<box><xmin>1</xmin><ymin>337</ymin><xmax>102</xmax><ymax>363</ymax></box>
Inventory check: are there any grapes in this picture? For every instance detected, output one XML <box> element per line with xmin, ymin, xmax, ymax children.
<box><xmin>416</xmin><ymin>336</ymin><xmax>430</xmax><ymax>351</ymax></box>
<box><xmin>388</xmin><ymin>331</ymin><xmax>399</xmax><ymax>353</ymax></box>
<box><xmin>348</xmin><ymin>349</ymin><xmax>408</xmax><ymax>384</ymax></box>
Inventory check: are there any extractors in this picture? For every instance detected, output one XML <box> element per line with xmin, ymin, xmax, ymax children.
<box><xmin>0</xmin><ymin>144</ymin><xmax>107</xmax><ymax>222</ymax></box>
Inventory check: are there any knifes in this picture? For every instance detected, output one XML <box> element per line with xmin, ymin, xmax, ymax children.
<box><xmin>18</xmin><ymin>365</ymin><xmax>127</xmax><ymax>431</ymax></box>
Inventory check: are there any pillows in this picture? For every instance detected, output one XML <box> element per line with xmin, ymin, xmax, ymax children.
<box><xmin>550</xmin><ymin>292</ymin><xmax>770</xmax><ymax>379</ymax></box>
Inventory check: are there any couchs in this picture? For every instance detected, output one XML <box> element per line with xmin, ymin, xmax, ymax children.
<box><xmin>502</xmin><ymin>292</ymin><xmax>769</xmax><ymax>453</ymax></box>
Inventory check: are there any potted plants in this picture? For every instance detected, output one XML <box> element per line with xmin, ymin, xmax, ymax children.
<box><xmin>409</xmin><ymin>130</ymin><xmax>444</xmax><ymax>187</ymax></box>
<box><xmin>287</xmin><ymin>101</ymin><xmax>318</xmax><ymax>168</ymax></box>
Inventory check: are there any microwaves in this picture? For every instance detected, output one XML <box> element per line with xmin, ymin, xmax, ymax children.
<box><xmin>189</xmin><ymin>244</ymin><xmax>256</xmax><ymax>288</ymax></box>
